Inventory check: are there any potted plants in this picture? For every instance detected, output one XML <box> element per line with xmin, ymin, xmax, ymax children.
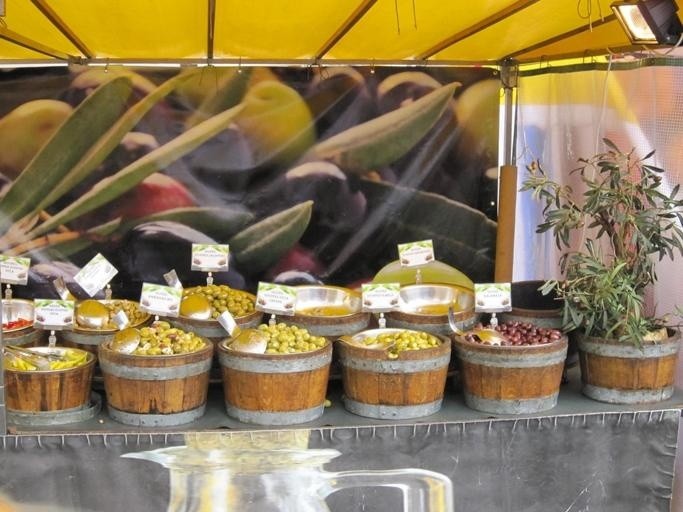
<box><xmin>513</xmin><ymin>134</ymin><xmax>681</xmax><ymax>403</ymax></box>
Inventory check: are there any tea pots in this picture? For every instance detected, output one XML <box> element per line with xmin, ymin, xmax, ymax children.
<box><xmin>120</xmin><ymin>446</ymin><xmax>453</xmax><ymax>511</ymax></box>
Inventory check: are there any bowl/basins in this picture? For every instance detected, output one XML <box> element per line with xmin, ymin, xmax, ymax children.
<box><xmin>397</xmin><ymin>282</ymin><xmax>474</xmax><ymax>317</ymax></box>
<box><xmin>292</xmin><ymin>285</ymin><xmax>366</xmax><ymax>319</ymax></box>
<box><xmin>0</xmin><ymin>298</ymin><xmax>39</xmax><ymax>337</ymax></box>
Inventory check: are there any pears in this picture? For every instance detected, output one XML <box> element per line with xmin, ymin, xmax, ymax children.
<box><xmin>0</xmin><ymin>99</ymin><xmax>74</xmax><ymax>174</ymax></box>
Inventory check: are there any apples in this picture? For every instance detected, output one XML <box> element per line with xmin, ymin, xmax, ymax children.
<box><xmin>456</xmin><ymin>77</ymin><xmax>503</xmax><ymax>172</ymax></box>
<box><xmin>276</xmin><ymin>247</ymin><xmax>322</xmax><ymax>273</ymax></box>
<box><xmin>107</xmin><ymin>172</ymin><xmax>200</xmax><ymax>224</ymax></box>
<box><xmin>234</xmin><ymin>81</ymin><xmax>317</xmax><ymax>164</ymax></box>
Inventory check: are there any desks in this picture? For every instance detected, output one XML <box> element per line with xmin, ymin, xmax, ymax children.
<box><xmin>0</xmin><ymin>367</ymin><xmax>682</xmax><ymax>512</ymax></box>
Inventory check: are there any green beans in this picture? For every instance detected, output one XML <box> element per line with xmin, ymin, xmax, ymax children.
<box><xmin>324</xmin><ymin>399</ymin><xmax>332</xmax><ymax>408</ymax></box>
<box><xmin>103</xmin><ymin>299</ymin><xmax>142</xmax><ymax>329</ymax></box>
<box><xmin>134</xmin><ymin>320</ymin><xmax>206</xmax><ymax>355</ymax></box>
<box><xmin>231</xmin><ymin>323</ymin><xmax>325</xmax><ymax>356</ymax></box>
<box><xmin>465</xmin><ymin>320</ymin><xmax>563</xmax><ymax>348</ymax></box>
<box><xmin>187</xmin><ymin>284</ymin><xmax>255</xmax><ymax>319</ymax></box>
<box><xmin>358</xmin><ymin>329</ymin><xmax>440</xmax><ymax>359</ymax></box>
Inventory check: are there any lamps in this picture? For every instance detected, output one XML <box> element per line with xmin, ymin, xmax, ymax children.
<box><xmin>610</xmin><ymin>0</ymin><xmax>682</xmax><ymax>45</ymax></box>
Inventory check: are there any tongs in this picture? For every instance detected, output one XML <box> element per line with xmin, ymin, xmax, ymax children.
<box><xmin>3</xmin><ymin>345</ymin><xmax>66</xmax><ymax>369</ymax></box>
